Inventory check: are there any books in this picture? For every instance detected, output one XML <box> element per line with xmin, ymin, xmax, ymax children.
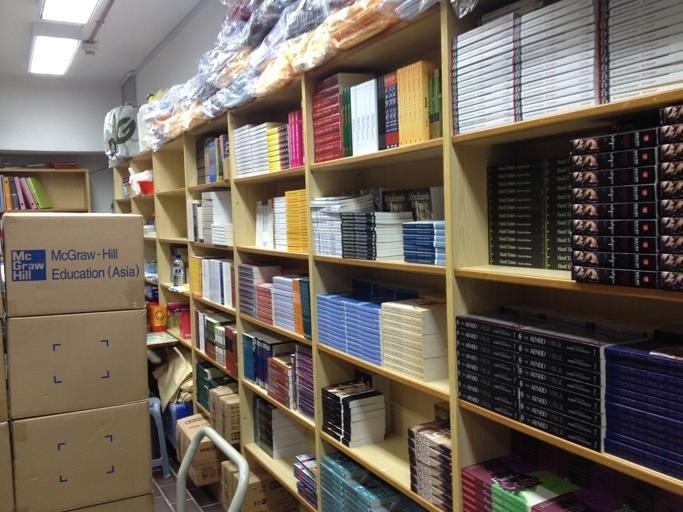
<box><xmin>456</xmin><ymin>303</ymin><xmax>682</xmax><ymax>480</ymax></box>
<box><xmin>0</xmin><ymin>172</ymin><xmax>51</xmax><ymax>211</ymax></box>
<box><xmin>313</xmin><ymin>0</ymin><xmax>682</xmax><ymax>164</ymax></box>
<box><xmin>197</xmin><ymin>344</ymin><xmax>454</xmax><ymax>512</ymax></box>
<box><xmin>204</xmin><ymin>101</ymin><xmax>305</xmax><ymax>184</ymax></box>
<box><xmin>489</xmin><ymin>105</ymin><xmax>682</xmax><ymax>290</ymax></box>
<box><xmin>461</xmin><ymin>455</ymin><xmax>683</xmax><ymax>512</ymax></box>
<box><xmin>188</xmin><ymin>187</ymin><xmax>449</xmax><ymax>382</ymax></box>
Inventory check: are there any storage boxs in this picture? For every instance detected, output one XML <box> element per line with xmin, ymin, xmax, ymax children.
<box><xmin>2</xmin><ymin>215</ymin><xmax>142</xmax><ymax>315</ymax></box>
<box><xmin>177</xmin><ymin>385</ymin><xmax>298</xmax><ymax>511</ymax></box>
<box><xmin>12</xmin><ymin>402</ymin><xmax>156</xmax><ymax>508</ymax></box>
<box><xmin>7</xmin><ymin>314</ymin><xmax>153</xmax><ymax>420</ymax></box>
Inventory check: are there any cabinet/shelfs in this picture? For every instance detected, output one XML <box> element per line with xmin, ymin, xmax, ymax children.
<box><xmin>300</xmin><ymin>2</ymin><xmax>454</xmax><ymax>511</ymax></box>
<box><xmin>110</xmin><ymin>131</ymin><xmax>194</xmax><ymax>459</ymax></box>
<box><xmin>184</xmin><ymin>79</ymin><xmax>318</xmax><ymax>511</ymax></box>
<box><xmin>1</xmin><ymin>171</ymin><xmax>90</xmax><ymax>211</ymax></box>
<box><xmin>443</xmin><ymin>4</ymin><xmax>682</xmax><ymax>510</ymax></box>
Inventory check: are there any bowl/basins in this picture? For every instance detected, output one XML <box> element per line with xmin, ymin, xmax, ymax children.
<box><xmin>137</xmin><ymin>180</ymin><xmax>153</xmax><ymax>195</ymax></box>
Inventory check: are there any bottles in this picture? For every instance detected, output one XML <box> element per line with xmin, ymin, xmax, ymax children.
<box><xmin>172</xmin><ymin>254</ymin><xmax>184</xmax><ymax>286</ymax></box>
